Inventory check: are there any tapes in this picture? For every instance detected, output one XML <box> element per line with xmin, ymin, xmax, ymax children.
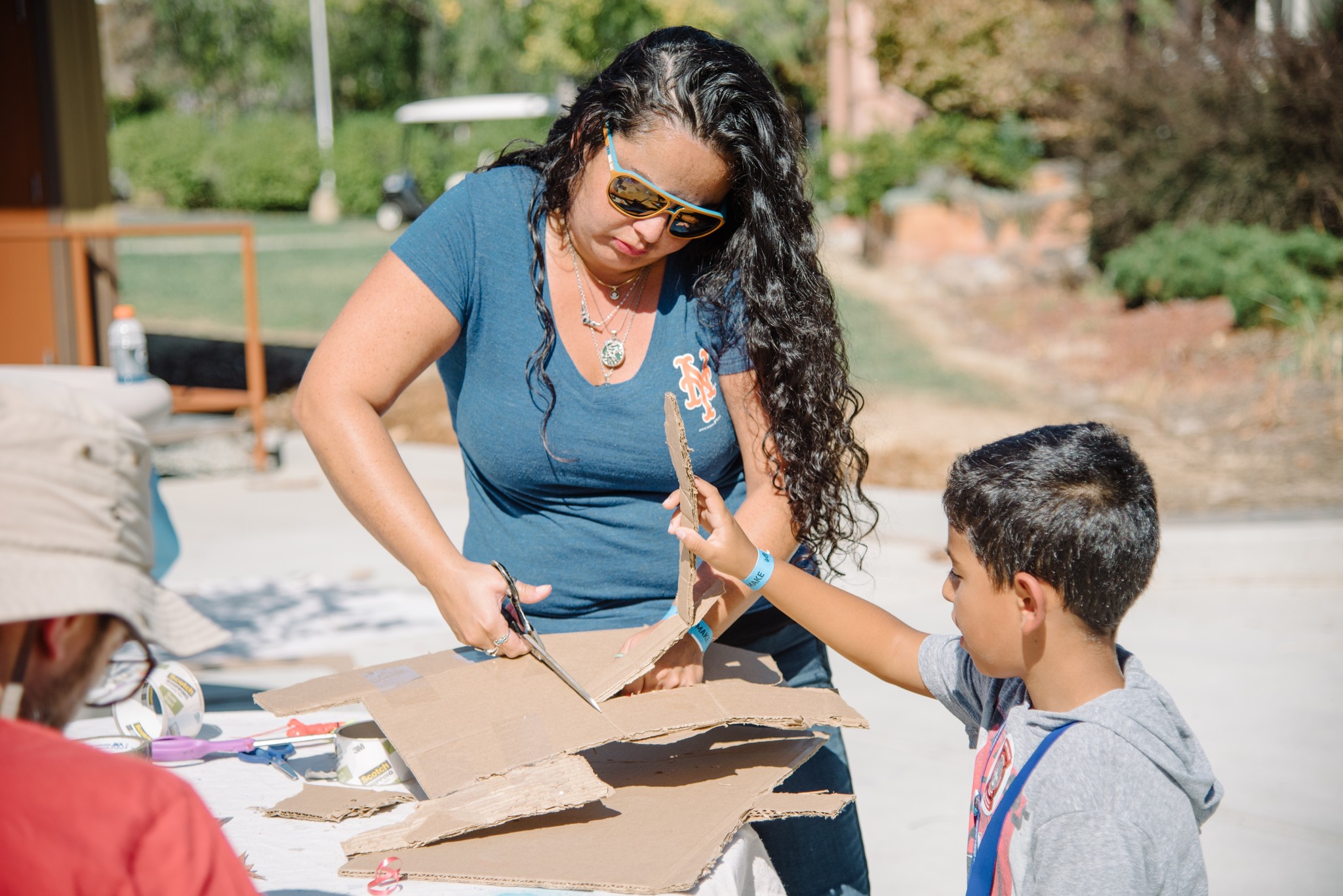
<box><xmin>70</xmin><ymin>735</ymin><xmax>152</xmax><ymax>764</ymax></box>
<box><xmin>111</xmin><ymin>660</ymin><xmax>205</xmax><ymax>741</ymax></box>
<box><xmin>335</xmin><ymin>720</ymin><xmax>415</xmax><ymax>786</ymax></box>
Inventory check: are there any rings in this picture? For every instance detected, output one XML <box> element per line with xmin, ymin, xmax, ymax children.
<box><xmin>474</xmin><ymin>647</ymin><xmax>485</xmax><ymax>652</ymax></box>
<box><xmin>494</xmin><ymin>628</ymin><xmax>510</xmax><ymax>646</ymax></box>
<box><xmin>485</xmin><ymin>647</ymin><xmax>497</xmax><ymax>656</ymax></box>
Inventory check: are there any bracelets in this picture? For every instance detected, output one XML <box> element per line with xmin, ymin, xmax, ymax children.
<box><xmin>664</xmin><ymin>606</ymin><xmax>713</xmax><ymax>654</ymax></box>
<box><xmin>742</xmin><ymin>549</ymin><xmax>774</xmax><ymax>592</ymax></box>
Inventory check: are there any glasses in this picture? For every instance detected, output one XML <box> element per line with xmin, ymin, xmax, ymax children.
<box><xmin>602</xmin><ymin>118</ymin><xmax>725</xmax><ymax>239</ymax></box>
<box><xmin>87</xmin><ymin>630</ymin><xmax>157</xmax><ymax>706</ymax></box>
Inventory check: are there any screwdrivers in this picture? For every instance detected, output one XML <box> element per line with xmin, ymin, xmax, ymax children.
<box><xmin>149</xmin><ymin>733</ymin><xmax>337</xmax><ymax>761</ymax></box>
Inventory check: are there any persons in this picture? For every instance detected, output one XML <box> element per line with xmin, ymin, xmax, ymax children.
<box><xmin>661</xmin><ymin>422</ymin><xmax>1230</xmax><ymax>896</ymax></box>
<box><xmin>289</xmin><ymin>28</ymin><xmax>874</xmax><ymax>896</ymax></box>
<box><xmin>0</xmin><ymin>360</ymin><xmax>265</xmax><ymax>896</ymax></box>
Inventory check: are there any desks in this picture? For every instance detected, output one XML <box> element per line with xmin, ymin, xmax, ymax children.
<box><xmin>146</xmin><ymin>710</ymin><xmax>788</xmax><ymax>896</ymax></box>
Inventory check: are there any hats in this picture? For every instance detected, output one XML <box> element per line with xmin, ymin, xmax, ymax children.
<box><xmin>0</xmin><ymin>381</ymin><xmax>230</xmax><ymax>657</ymax></box>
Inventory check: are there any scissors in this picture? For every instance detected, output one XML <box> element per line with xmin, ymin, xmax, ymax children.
<box><xmin>491</xmin><ymin>560</ymin><xmax>602</xmax><ymax>756</ymax></box>
<box><xmin>238</xmin><ymin>743</ymin><xmax>298</xmax><ymax>780</ymax></box>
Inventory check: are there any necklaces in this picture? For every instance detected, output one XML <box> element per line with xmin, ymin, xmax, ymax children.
<box><xmin>558</xmin><ymin>207</ymin><xmax>648</xmax><ymax>380</ymax></box>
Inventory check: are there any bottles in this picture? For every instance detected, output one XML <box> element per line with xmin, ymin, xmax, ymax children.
<box><xmin>108</xmin><ymin>304</ymin><xmax>148</xmax><ymax>382</ymax></box>
